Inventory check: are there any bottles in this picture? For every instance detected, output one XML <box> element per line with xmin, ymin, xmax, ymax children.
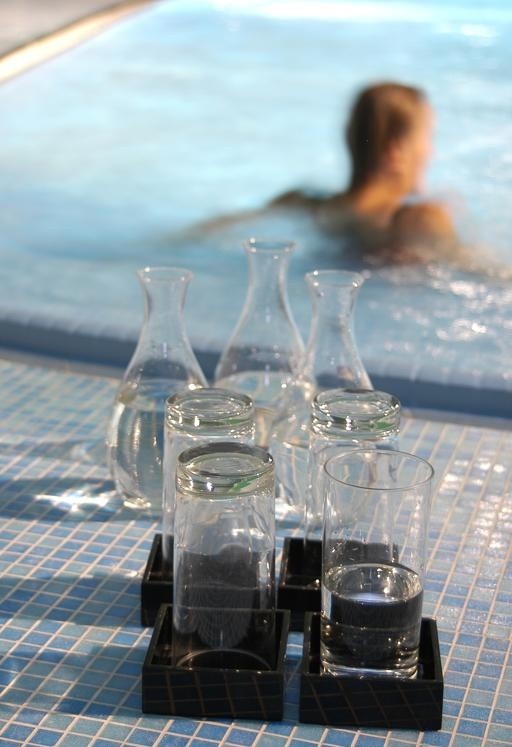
<box><xmin>207</xmin><ymin>236</ymin><xmax>308</xmax><ymax>454</ymax></box>
<box><xmin>258</xmin><ymin>269</ymin><xmax>377</xmax><ymax>531</ymax></box>
<box><xmin>100</xmin><ymin>265</ymin><xmax>209</xmax><ymax>515</ymax></box>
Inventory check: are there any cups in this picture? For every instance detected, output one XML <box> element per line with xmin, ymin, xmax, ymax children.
<box><xmin>305</xmin><ymin>389</ymin><xmax>400</xmax><ymax>590</ymax></box>
<box><xmin>172</xmin><ymin>441</ymin><xmax>276</xmax><ymax>668</ymax></box>
<box><xmin>321</xmin><ymin>449</ymin><xmax>435</xmax><ymax>678</ymax></box>
<box><xmin>161</xmin><ymin>388</ymin><xmax>256</xmax><ymax>584</ymax></box>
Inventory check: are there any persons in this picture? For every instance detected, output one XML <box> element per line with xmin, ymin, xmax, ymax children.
<box><xmin>183</xmin><ymin>83</ymin><xmax>512</xmax><ymax>280</ymax></box>
